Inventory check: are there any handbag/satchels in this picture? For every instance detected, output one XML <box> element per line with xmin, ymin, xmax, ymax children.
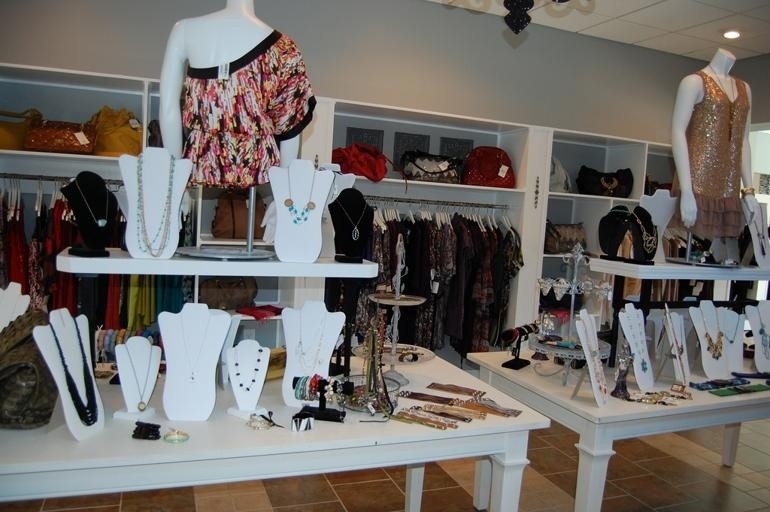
<box><xmin>201</xmin><ymin>275</ymin><xmax>258</xmax><ymax>311</ymax></box>
<box><xmin>544</xmin><ymin>215</ymin><xmax>587</xmax><ymax>254</ymax></box>
<box><xmin>577</xmin><ymin>165</ymin><xmax>633</xmax><ymax>197</ymax></box>
<box><xmin>332</xmin><ymin>145</ymin><xmax>515</xmax><ymax>188</ymax></box>
<box><xmin>211</xmin><ymin>188</ymin><xmax>267</xmax><ymax>239</ymax></box>
<box><xmin>0</xmin><ymin>104</ymin><xmax>144</xmax><ymax>158</ymax></box>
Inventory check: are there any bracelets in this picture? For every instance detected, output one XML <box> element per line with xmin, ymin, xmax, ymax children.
<box><xmin>741</xmin><ymin>187</ymin><xmax>755</xmax><ymax>197</ymax></box>
<box><xmin>293</xmin><ymin>373</ymin><xmax>323</xmax><ymax>401</ymax></box>
<box><xmin>164</xmin><ymin>430</ymin><xmax>189</xmax><ymax>444</ymax></box>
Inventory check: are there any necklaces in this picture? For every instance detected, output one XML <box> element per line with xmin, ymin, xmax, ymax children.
<box><xmin>579</xmin><ymin>317</ymin><xmax>608</xmax><ymax>394</ymax></box>
<box><xmin>708</xmin><ymin>64</ymin><xmax>736</xmax><ymax>126</ymax></box>
<box><xmin>337</xmin><ymin>198</ymin><xmax>367</xmax><ymax>241</ymax></box>
<box><xmin>136</xmin><ymin>153</ymin><xmax>176</xmax><ymax>257</ymax></box>
<box><xmin>299</xmin><ymin>307</ymin><xmax>328</xmax><ymax>373</ymax></box>
<box><xmin>632</xmin><ymin>210</ymin><xmax>658</xmax><ymax>254</ymax></box>
<box><xmin>75</xmin><ymin>178</ymin><xmax>111</xmax><ymax>228</ymax></box>
<box><xmin>45</xmin><ymin>316</ymin><xmax>98</xmax><ymax>427</ymax></box>
<box><xmin>626</xmin><ymin>311</ymin><xmax>647</xmax><ymax>373</ymax></box>
<box><xmin>741</xmin><ymin>198</ymin><xmax>767</xmax><ymax>258</ymax></box>
<box><xmin>233</xmin><ymin>343</ymin><xmax>264</xmax><ymax>392</ymax></box>
<box><xmin>124</xmin><ymin>343</ymin><xmax>153</xmax><ymax>411</ymax></box>
<box><xmin>284</xmin><ymin>164</ymin><xmax>316</xmax><ymax>225</ymax></box>
<box><xmin>698</xmin><ymin>306</ymin><xmax>739</xmax><ymax>361</ymax></box>
<box><xmin>179</xmin><ymin>310</ymin><xmax>212</xmax><ymax>381</ymax></box>
<box><xmin>756</xmin><ymin>305</ymin><xmax>770</xmax><ymax>361</ymax></box>
<box><xmin>669</xmin><ymin>315</ymin><xmax>683</xmax><ymax>354</ymax></box>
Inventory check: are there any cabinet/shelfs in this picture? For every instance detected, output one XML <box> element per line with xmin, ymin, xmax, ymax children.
<box><xmin>0</xmin><ymin>76</ymin><xmax>532</xmax><ymax>364</ymax></box>
<box><xmin>1</xmin><ymin>343</ymin><xmax>551</xmax><ymax>512</ymax></box>
<box><xmin>467</xmin><ymin>331</ymin><xmax>770</xmax><ymax>511</ymax></box>
<box><xmin>540</xmin><ymin>126</ymin><xmax>706</xmax><ymax>327</ymax></box>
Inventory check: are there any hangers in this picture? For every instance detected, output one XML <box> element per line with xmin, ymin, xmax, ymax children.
<box><xmin>361</xmin><ymin>193</ymin><xmax>522</xmax><ymax>245</ymax></box>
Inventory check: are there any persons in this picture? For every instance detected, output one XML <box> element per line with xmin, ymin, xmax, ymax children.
<box><xmin>672</xmin><ymin>48</ymin><xmax>761</xmax><ymax>229</ymax></box>
<box><xmin>159</xmin><ymin>0</ymin><xmax>316</xmax><ymax>244</ymax></box>
